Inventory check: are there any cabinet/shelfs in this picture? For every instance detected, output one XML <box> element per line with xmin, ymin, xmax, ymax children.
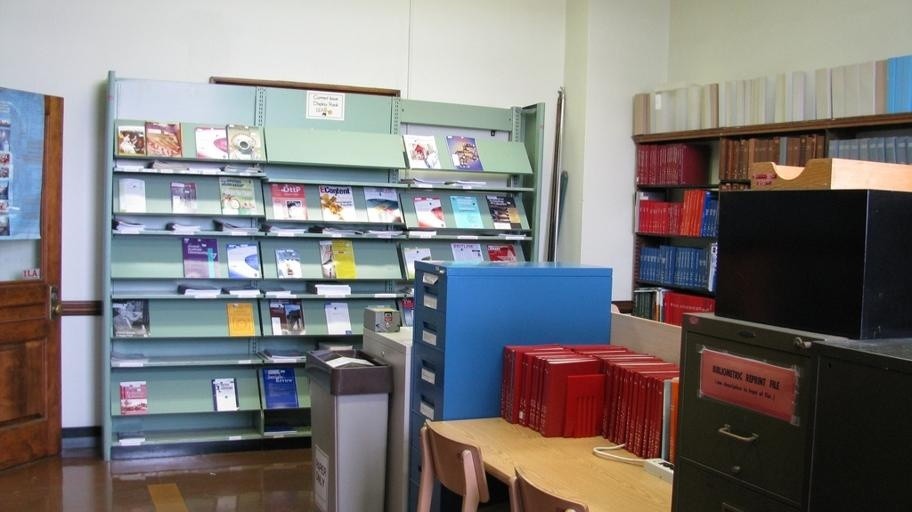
<box><xmin>630</xmin><ymin>110</ymin><xmax>910</xmax><ymax>328</ymax></box>
<box><xmin>669</xmin><ymin>311</ymin><xmax>848</xmax><ymax>512</ymax></box>
<box><xmin>99</xmin><ymin>70</ymin><xmax>546</xmax><ymax>463</ymax></box>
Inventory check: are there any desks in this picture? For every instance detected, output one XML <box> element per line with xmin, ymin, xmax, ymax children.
<box><xmin>430</xmin><ymin>416</ymin><xmax>675</xmax><ymax>512</ymax></box>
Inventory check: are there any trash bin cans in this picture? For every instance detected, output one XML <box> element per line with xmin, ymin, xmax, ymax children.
<box><xmin>305</xmin><ymin>351</ymin><xmax>392</xmax><ymax>511</ymax></box>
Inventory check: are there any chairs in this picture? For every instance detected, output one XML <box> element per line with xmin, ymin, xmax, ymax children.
<box><xmin>415</xmin><ymin>418</ymin><xmax>590</xmax><ymax>512</ymax></box>
<box><xmin>407</xmin><ymin>258</ymin><xmax>613</xmax><ymax>511</ymax></box>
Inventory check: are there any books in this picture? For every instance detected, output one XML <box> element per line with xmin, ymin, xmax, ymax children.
<box><xmin>630</xmin><ymin>55</ymin><xmax>912</xmax><ymax>329</ymax></box>
<box><xmin>499</xmin><ymin>336</ymin><xmax>680</xmax><ymax>467</ymax></box>
<box><xmin>403</xmin><ymin>133</ymin><xmax>525</xmax><ymax>327</ymax></box>
<box><xmin>262</xmin><ymin>183</ymin><xmax>404</xmax><ymax>411</ymax></box>
<box><xmin>112</xmin><ymin>123</ymin><xmax>269</xmax><ymax>411</ymax></box>
